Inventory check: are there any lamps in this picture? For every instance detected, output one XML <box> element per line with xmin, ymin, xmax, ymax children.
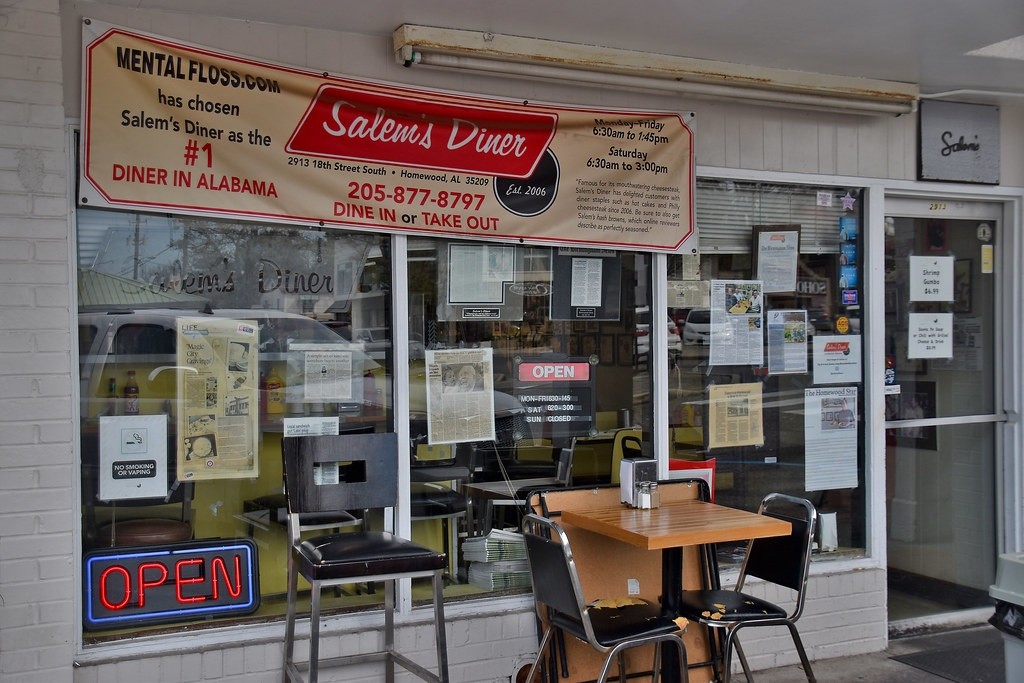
<box><xmin>393</xmin><ymin>23</ymin><xmax>921</xmax><ymax>117</ymax></box>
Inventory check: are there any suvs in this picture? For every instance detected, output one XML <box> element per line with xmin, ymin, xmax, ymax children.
<box><xmin>71</xmin><ymin>301</ymin><xmax>527</xmax><ymax>444</ymax></box>
<box><xmin>617</xmin><ymin>300</ymin><xmax>870</xmax><ymax>364</ymax></box>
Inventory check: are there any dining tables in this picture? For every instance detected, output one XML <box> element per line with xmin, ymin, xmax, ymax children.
<box><xmin>561</xmin><ymin>499</ymin><xmax>794</xmax><ymax>683</ymax></box>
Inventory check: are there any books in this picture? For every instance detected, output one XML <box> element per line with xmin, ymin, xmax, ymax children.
<box><xmin>461</xmin><ymin>527</ymin><xmax>534</xmax><ymax>592</ymax></box>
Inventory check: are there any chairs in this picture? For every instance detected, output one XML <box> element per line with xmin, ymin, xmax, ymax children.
<box><xmin>652</xmin><ymin>492</ymin><xmax>818</xmax><ymax>683</ymax></box>
<box><xmin>244</xmin><ymin>426</ymin><xmax>376</xmax><ymax>597</ymax></box>
<box><xmin>520</xmin><ymin>515</ymin><xmax>690</xmax><ymax>683</ymax></box>
<box><xmin>281</xmin><ymin>434</ymin><xmax>452</xmax><ymax>683</ymax></box>
<box><xmin>410</xmin><ymin>490</ymin><xmax>469</xmax><ymax>588</ymax></box>
<box><xmin>83</xmin><ymin>433</ymin><xmax>196</xmax><ymax>547</ymax></box>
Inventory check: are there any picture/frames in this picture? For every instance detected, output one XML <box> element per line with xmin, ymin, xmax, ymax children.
<box><xmin>885</xmin><ymin>379</ymin><xmax>938</xmax><ymax>450</ymax></box>
<box><xmin>939</xmin><ymin>258</ymin><xmax>971</xmax><ymax>314</ymax></box>
<box><xmin>890</xmin><ymin>328</ymin><xmax>927</xmax><ymax>376</ymax></box>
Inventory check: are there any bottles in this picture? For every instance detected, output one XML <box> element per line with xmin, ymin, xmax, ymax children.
<box><xmin>635</xmin><ymin>479</ymin><xmax>660</xmax><ymax>510</ymax></box>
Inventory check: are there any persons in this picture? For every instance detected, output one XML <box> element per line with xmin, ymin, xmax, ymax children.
<box><xmin>726</xmin><ymin>288</ymin><xmax>745</xmax><ymax>313</ymax></box>
<box><xmin>790</xmin><ymin>327</ymin><xmax>801</xmax><ymax>342</ymax></box>
<box><xmin>748</xmin><ymin>290</ymin><xmax>761</xmax><ymax>313</ymax></box>
<box><xmin>458</xmin><ymin>365</ymin><xmax>483</xmax><ymax>392</ymax></box>
<box><xmin>441</xmin><ymin>365</ymin><xmax>459</xmax><ymax>394</ymax></box>
